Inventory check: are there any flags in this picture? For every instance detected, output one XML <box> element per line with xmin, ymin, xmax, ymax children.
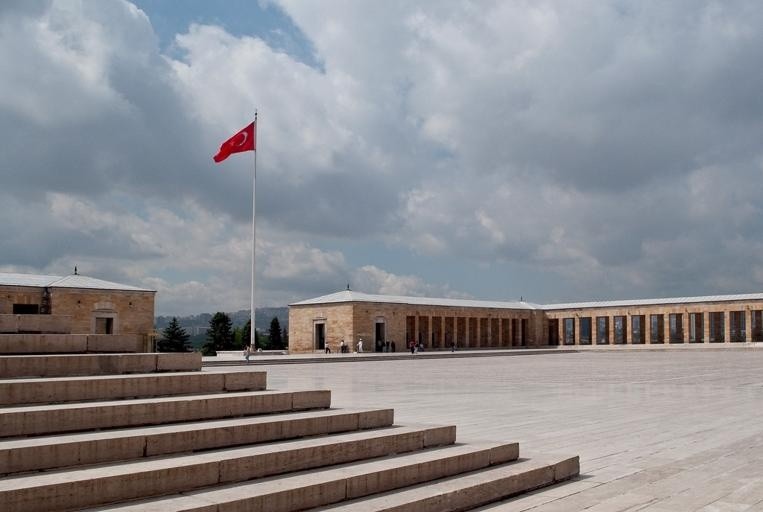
<box><xmin>212</xmin><ymin>121</ymin><xmax>254</xmax><ymax>163</ymax></box>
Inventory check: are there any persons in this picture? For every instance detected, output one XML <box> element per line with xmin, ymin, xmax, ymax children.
<box><xmin>335</xmin><ymin>340</ymin><xmax>339</xmax><ymax>353</ymax></box>
<box><xmin>385</xmin><ymin>339</ymin><xmax>390</xmax><ymax>352</ymax></box>
<box><xmin>340</xmin><ymin>339</ymin><xmax>343</xmax><ymax>353</ymax></box>
<box><xmin>413</xmin><ymin>341</ymin><xmax>419</xmax><ymax>353</ymax></box>
<box><xmin>419</xmin><ymin>342</ymin><xmax>424</xmax><ymax>351</ymax></box>
<box><xmin>357</xmin><ymin>338</ymin><xmax>363</xmax><ymax>352</ymax></box>
<box><xmin>325</xmin><ymin>341</ymin><xmax>331</xmax><ymax>354</ymax></box>
<box><xmin>244</xmin><ymin>344</ymin><xmax>249</xmax><ymax>363</ymax></box>
<box><xmin>409</xmin><ymin>340</ymin><xmax>415</xmax><ymax>354</ymax></box>
<box><xmin>390</xmin><ymin>340</ymin><xmax>395</xmax><ymax>352</ymax></box>
<box><xmin>381</xmin><ymin>340</ymin><xmax>386</xmax><ymax>352</ymax></box>
<box><xmin>449</xmin><ymin>341</ymin><xmax>454</xmax><ymax>352</ymax></box>
